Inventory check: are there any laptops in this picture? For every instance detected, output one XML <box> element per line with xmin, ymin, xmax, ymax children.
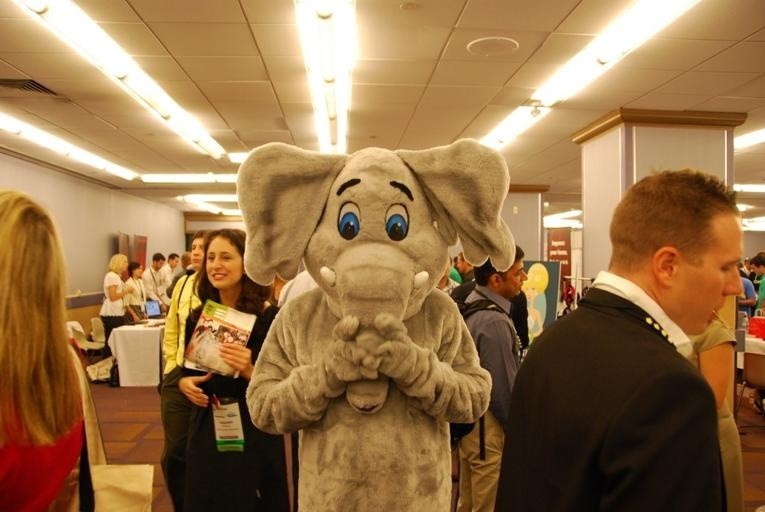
<box><xmin>145</xmin><ymin>300</ymin><xmax>165</xmax><ymax>318</ymax></box>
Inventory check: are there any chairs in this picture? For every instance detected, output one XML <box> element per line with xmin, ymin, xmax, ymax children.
<box><xmin>89</xmin><ymin>317</ymin><xmax>105</xmax><ymax>344</ymax></box>
<box><xmin>66</xmin><ymin>321</ymin><xmax>105</xmax><ymax>365</ymax></box>
<box><xmin>735</xmin><ymin>351</ymin><xmax>764</xmax><ymax>415</ymax></box>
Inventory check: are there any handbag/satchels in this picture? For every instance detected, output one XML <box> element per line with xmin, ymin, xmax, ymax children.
<box><xmin>109</xmin><ymin>364</ymin><xmax>119</xmax><ymax>386</ymax></box>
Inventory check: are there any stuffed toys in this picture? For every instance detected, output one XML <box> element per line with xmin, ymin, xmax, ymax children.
<box><xmin>235</xmin><ymin>140</ymin><xmax>516</xmax><ymax>512</ymax></box>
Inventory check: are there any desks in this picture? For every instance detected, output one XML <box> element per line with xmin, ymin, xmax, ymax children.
<box><xmin>735</xmin><ymin>328</ymin><xmax>765</xmax><ymax>370</ymax></box>
<box><xmin>106</xmin><ymin>316</ymin><xmax>166</xmax><ymax>390</ymax></box>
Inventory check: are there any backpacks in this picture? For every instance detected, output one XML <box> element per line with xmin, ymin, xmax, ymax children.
<box><xmin>448</xmin><ymin>281</ymin><xmax>521</xmax><ymax>436</ymax></box>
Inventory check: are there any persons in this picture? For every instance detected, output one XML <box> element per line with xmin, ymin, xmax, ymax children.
<box><xmin>436</xmin><ymin>245</ymin><xmax>528</xmax><ymax>510</ymax></box>
<box><xmin>163</xmin><ymin>227</ymin><xmax>299</xmax><ymax>512</ymax></box>
<box><xmin>99</xmin><ymin>229</ymin><xmax>300</xmax><ymax>436</ymax></box>
<box><xmin>0</xmin><ymin>189</ymin><xmax>84</xmax><ymax>512</ymax></box>
<box><xmin>498</xmin><ymin>169</ymin><xmax>764</xmax><ymax>511</ymax></box>
